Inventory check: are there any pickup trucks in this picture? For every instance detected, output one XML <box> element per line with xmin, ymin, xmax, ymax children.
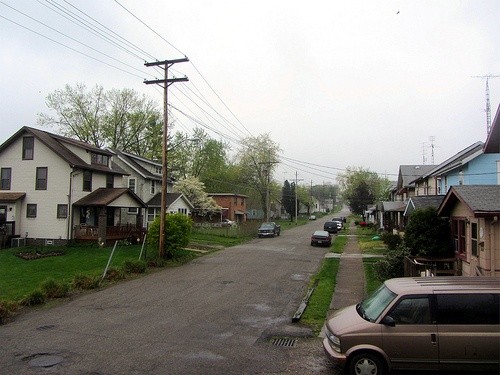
<box><xmin>258</xmin><ymin>222</ymin><xmax>280</xmax><ymax>238</ymax></box>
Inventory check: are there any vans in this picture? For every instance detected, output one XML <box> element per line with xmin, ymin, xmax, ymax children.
<box><xmin>323</xmin><ymin>276</ymin><xmax>500</xmax><ymax>375</ymax></box>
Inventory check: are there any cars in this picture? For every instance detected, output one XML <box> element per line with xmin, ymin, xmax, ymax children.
<box><xmin>324</xmin><ymin>222</ymin><xmax>338</xmax><ymax>233</ymax></box>
<box><xmin>332</xmin><ymin>217</ymin><xmax>342</xmax><ymax>225</ymax></box>
<box><xmin>331</xmin><ymin>220</ymin><xmax>343</xmax><ymax>231</ymax></box>
<box><xmin>311</xmin><ymin>230</ymin><xmax>332</xmax><ymax>247</ymax></box>
